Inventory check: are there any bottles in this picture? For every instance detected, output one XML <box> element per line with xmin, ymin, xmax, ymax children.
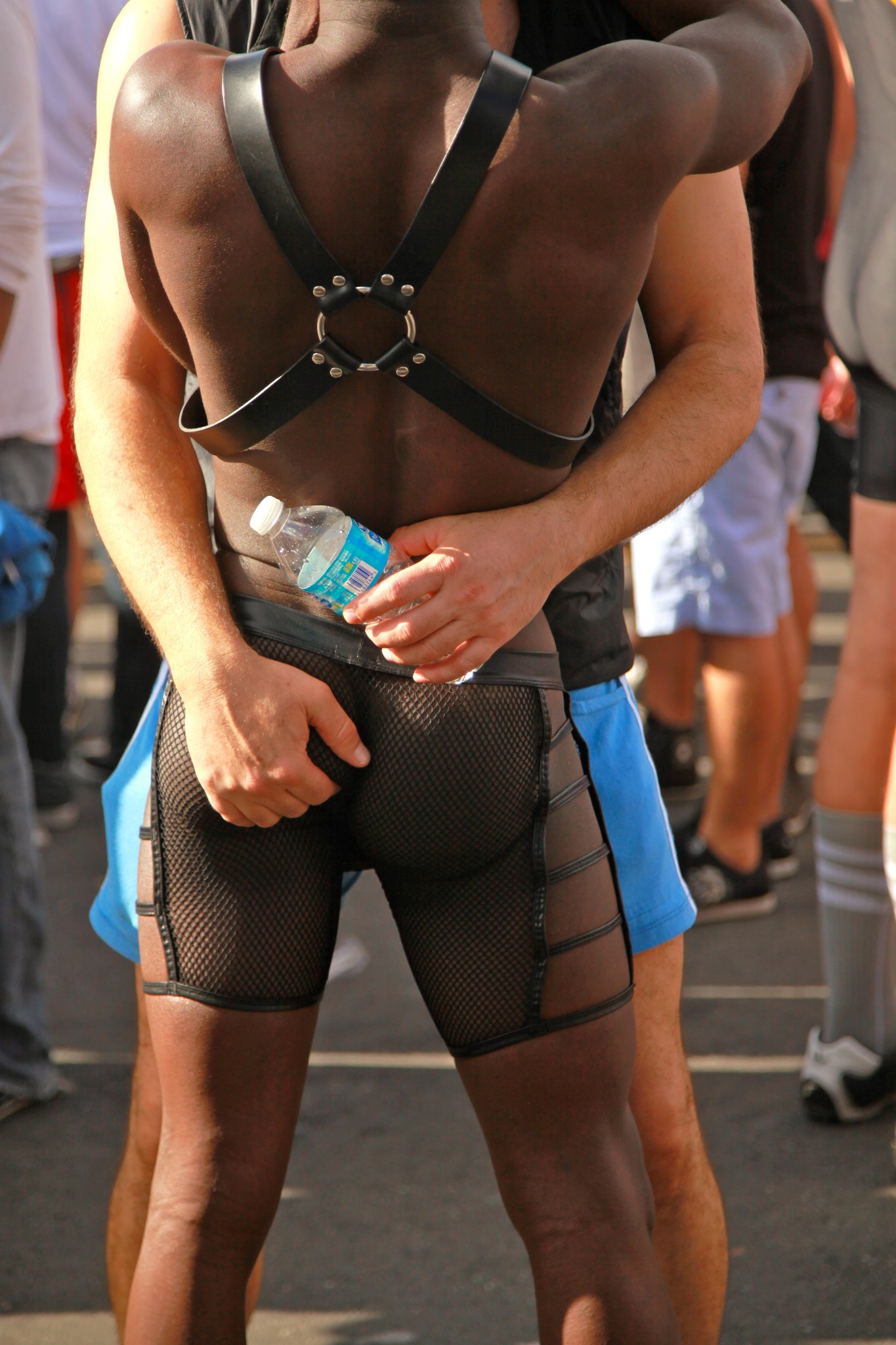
<box><xmin>249</xmin><ymin>496</ymin><xmax>486</xmax><ymax>685</ymax></box>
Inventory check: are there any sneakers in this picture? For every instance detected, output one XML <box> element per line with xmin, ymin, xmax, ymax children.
<box><xmin>800</xmin><ymin>1027</ymin><xmax>896</xmax><ymax>1127</ymax></box>
<box><xmin>670</xmin><ymin>811</ymin><xmax>801</xmax><ymax>925</ymax></box>
<box><xmin>634</xmin><ymin>699</ymin><xmax>702</xmax><ymax>802</ymax></box>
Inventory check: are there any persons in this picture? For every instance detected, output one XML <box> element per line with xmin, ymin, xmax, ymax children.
<box><xmin>0</xmin><ymin>0</ymin><xmax>896</xmax><ymax>1345</ymax></box>
